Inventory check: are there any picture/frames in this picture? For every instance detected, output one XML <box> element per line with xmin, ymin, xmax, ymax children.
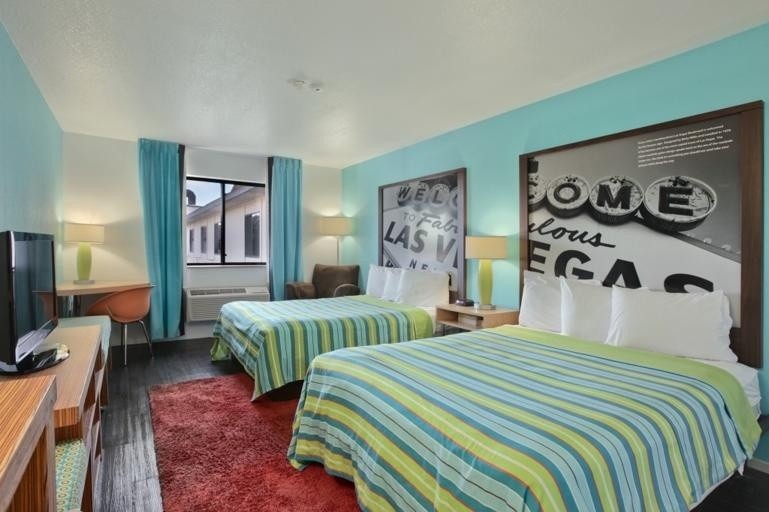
<box><xmin>518</xmin><ymin>100</ymin><xmax>763</xmax><ymax>369</ymax></box>
<box><xmin>377</xmin><ymin>167</ymin><xmax>467</xmax><ymax>304</ymax></box>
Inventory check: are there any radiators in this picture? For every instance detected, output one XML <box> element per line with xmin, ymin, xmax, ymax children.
<box><xmin>183</xmin><ymin>286</ymin><xmax>270</xmax><ymax>323</ymax></box>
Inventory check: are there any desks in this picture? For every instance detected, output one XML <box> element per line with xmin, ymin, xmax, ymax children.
<box><xmin>0</xmin><ymin>315</ymin><xmax>111</xmax><ymax>512</ymax></box>
<box><xmin>55</xmin><ymin>280</ymin><xmax>151</xmax><ymax>317</ymax></box>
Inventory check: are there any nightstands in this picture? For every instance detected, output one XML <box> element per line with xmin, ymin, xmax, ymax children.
<box><xmin>435</xmin><ymin>304</ymin><xmax>519</xmax><ymax>336</ymax></box>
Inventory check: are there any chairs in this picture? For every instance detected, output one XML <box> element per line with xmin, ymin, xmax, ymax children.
<box><xmin>85</xmin><ymin>284</ymin><xmax>155</xmax><ymax>367</ymax></box>
<box><xmin>286</xmin><ymin>264</ymin><xmax>360</xmax><ymax>300</ymax></box>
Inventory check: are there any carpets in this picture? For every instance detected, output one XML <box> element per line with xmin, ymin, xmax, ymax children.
<box><xmin>147</xmin><ymin>372</ymin><xmax>362</xmax><ymax>512</ymax></box>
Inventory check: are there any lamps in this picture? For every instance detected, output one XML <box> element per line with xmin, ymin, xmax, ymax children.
<box><xmin>465</xmin><ymin>235</ymin><xmax>506</xmax><ymax>309</ymax></box>
<box><xmin>63</xmin><ymin>221</ymin><xmax>104</xmax><ymax>284</ymax></box>
<box><xmin>320</xmin><ymin>216</ymin><xmax>352</xmax><ymax>265</ymax></box>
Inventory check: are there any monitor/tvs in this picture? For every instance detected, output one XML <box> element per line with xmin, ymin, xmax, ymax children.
<box><xmin>0</xmin><ymin>230</ymin><xmax>70</xmax><ymax>376</ymax></box>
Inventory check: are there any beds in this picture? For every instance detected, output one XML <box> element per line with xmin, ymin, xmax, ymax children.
<box><xmin>287</xmin><ymin>325</ymin><xmax>762</xmax><ymax>512</ymax></box>
<box><xmin>210</xmin><ymin>294</ymin><xmax>436</xmax><ymax>404</ymax></box>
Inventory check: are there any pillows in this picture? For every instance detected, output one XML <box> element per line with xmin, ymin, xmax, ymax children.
<box><xmin>519</xmin><ymin>269</ymin><xmax>602</xmax><ymax>333</ymax></box>
<box><xmin>604</xmin><ymin>284</ymin><xmax>739</xmax><ymax>362</ymax></box>
<box><xmin>365</xmin><ymin>264</ymin><xmax>388</xmax><ymax>298</ymax></box>
<box><xmin>559</xmin><ymin>275</ymin><xmax>650</xmax><ymax>344</ymax></box>
<box><xmin>380</xmin><ymin>268</ymin><xmax>403</xmax><ymax>301</ymax></box>
<box><xmin>395</xmin><ymin>269</ymin><xmax>450</xmax><ymax>309</ymax></box>
